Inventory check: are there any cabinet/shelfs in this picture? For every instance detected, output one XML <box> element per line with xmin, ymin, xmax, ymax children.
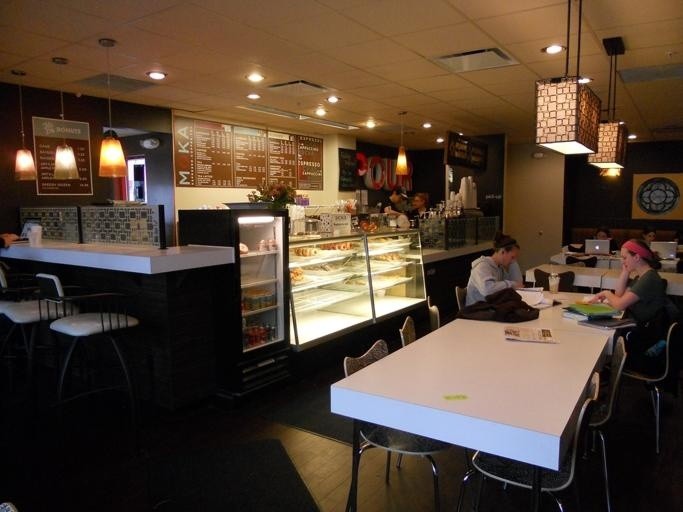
<box><xmin>288</xmin><ymin>229</ymin><xmax>427</xmax><ymax>353</ymax></box>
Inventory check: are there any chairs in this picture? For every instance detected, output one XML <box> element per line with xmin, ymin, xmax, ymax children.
<box><xmin>0</xmin><ymin>268</ymin><xmax>79</xmax><ymax>394</ymax></box>
<box><xmin>35</xmin><ymin>273</ymin><xmax>140</xmax><ymax>408</ymax></box>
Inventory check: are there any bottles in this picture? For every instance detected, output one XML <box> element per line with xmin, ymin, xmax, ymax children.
<box><xmin>242</xmin><ymin>287</ymin><xmax>276</xmax><ymax>309</ymax></box>
<box><xmin>410</xmin><ymin>192</ymin><xmax>465</xmax><ymax>249</ymax></box>
<box><xmin>243</xmin><ymin>318</ymin><xmax>275</xmax><ymax>349</ymax></box>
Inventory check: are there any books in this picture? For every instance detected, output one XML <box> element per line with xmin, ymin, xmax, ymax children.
<box><xmin>580</xmin><ymin>318</ymin><xmax>637</xmax><ymax>329</ymax></box>
<box><xmin>562</xmin><ymin>303</ymin><xmax>617</xmax><ymax>319</ymax></box>
<box><xmin>515</xmin><ymin>286</ymin><xmax>562</xmax><ymax>310</ymax></box>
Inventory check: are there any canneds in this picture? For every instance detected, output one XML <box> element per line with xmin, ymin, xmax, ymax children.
<box><xmin>243</xmin><ymin>290</ymin><xmax>275</xmax><ymax>311</ymax></box>
<box><xmin>246</xmin><ymin>317</ymin><xmax>277</xmax><ymax>350</ymax></box>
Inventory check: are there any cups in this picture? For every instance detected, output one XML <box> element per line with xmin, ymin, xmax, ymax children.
<box><xmin>28</xmin><ymin>226</ymin><xmax>42</xmax><ymax>245</ymax></box>
<box><xmin>452</xmin><ymin>175</ymin><xmax>477</xmax><ymax>209</ymax></box>
<box><xmin>549</xmin><ymin>274</ymin><xmax>560</xmax><ymax>293</ymax></box>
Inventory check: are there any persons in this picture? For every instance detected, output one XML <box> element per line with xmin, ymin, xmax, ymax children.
<box><xmin>464</xmin><ymin>230</ymin><xmax>526</xmax><ymax>307</ymax></box>
<box><xmin>640</xmin><ymin>225</ymin><xmax>658</xmax><ymax>244</ymax></box>
<box><xmin>0</xmin><ymin>232</ymin><xmax>20</xmax><ymax>249</ymax></box>
<box><xmin>380</xmin><ymin>185</ymin><xmax>408</xmax><ymax>216</ymax></box>
<box><xmin>583</xmin><ymin>238</ymin><xmax>667</xmax><ymax>378</ymax></box>
<box><xmin>581</xmin><ymin>226</ymin><xmax>619</xmax><ymax>253</ymax></box>
<box><xmin>411</xmin><ymin>192</ymin><xmax>430</xmax><ymax>228</ymax></box>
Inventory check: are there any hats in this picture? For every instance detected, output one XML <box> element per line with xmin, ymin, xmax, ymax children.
<box><xmin>393</xmin><ymin>185</ymin><xmax>408</xmax><ymax>199</ymax></box>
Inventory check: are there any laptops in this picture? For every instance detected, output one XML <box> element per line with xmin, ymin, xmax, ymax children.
<box><xmin>650</xmin><ymin>242</ymin><xmax>677</xmax><ymax>260</ymax></box>
<box><xmin>11</xmin><ymin>217</ymin><xmax>41</xmax><ymax>244</ymax></box>
<box><xmin>585</xmin><ymin>239</ymin><xmax>610</xmax><ymax>255</ymax></box>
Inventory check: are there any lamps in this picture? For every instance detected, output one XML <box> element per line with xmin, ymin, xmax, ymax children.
<box><xmin>535</xmin><ymin>1</ymin><xmax>602</xmax><ymax>155</ymax></box>
<box><xmin>52</xmin><ymin>57</ymin><xmax>80</xmax><ymax>180</ymax></box>
<box><xmin>98</xmin><ymin>38</ymin><xmax>128</xmax><ymax>178</ymax></box>
<box><xmin>588</xmin><ymin>36</ymin><xmax>628</xmax><ymax>169</ymax></box>
<box><xmin>12</xmin><ymin>69</ymin><xmax>37</xmax><ymax>182</ymax></box>
<box><xmin>394</xmin><ymin>112</ymin><xmax>408</xmax><ymax>175</ymax></box>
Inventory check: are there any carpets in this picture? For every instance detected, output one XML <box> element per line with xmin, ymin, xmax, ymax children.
<box><xmin>258</xmin><ymin>383</ymin><xmax>368</xmax><ymax>446</ymax></box>
<box><xmin>147</xmin><ymin>439</ymin><xmax>321</xmax><ymax>512</ymax></box>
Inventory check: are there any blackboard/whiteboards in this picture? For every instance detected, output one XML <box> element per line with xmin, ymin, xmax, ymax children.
<box><xmin>338</xmin><ymin>147</ymin><xmax>357</xmax><ymax>192</ymax></box>
